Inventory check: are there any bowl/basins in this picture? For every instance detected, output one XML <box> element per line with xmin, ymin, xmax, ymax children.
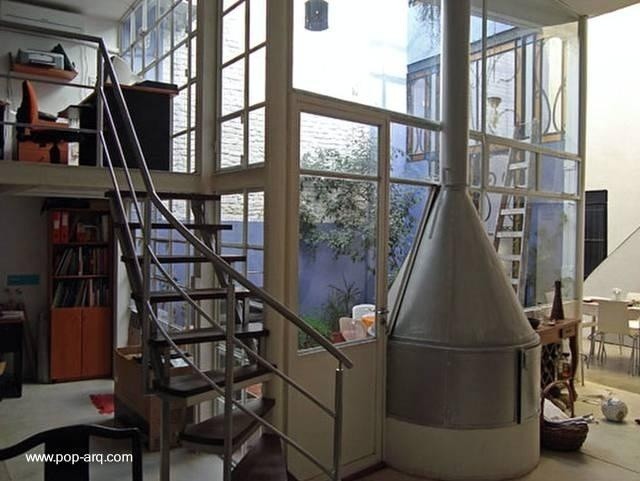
<box><xmin>528</xmin><ymin>317</ymin><xmax>539</xmax><ymax>330</ymax></box>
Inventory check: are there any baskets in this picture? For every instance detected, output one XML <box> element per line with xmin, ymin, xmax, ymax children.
<box><xmin>541</xmin><ymin>380</ymin><xmax>589</xmax><ymax>452</ymax></box>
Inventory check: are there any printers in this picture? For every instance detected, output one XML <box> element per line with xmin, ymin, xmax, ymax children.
<box><xmin>15</xmin><ymin>47</ymin><xmax>65</xmax><ymax>70</ymax></box>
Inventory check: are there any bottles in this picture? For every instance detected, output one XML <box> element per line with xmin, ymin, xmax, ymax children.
<box><xmin>535</xmin><ymin>301</ymin><xmax>544</xmax><ymax>327</ymax></box>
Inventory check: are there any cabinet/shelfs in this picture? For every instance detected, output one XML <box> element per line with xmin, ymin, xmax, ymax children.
<box><xmin>42</xmin><ymin>196</ymin><xmax>117</xmax><ymax>385</ymax></box>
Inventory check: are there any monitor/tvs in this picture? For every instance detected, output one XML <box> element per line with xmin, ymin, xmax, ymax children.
<box><xmin>95</xmin><ymin>55</ymin><xmax>143</xmax><ymax>86</ymax></box>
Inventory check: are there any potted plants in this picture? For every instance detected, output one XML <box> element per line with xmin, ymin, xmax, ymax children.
<box><xmin>325</xmin><ymin>298</ymin><xmax>351</xmax><ymax>343</ymax></box>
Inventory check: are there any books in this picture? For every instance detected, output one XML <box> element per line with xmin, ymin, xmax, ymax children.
<box><xmin>51</xmin><ymin>209</ymin><xmax>114</xmax><ymax>309</ymax></box>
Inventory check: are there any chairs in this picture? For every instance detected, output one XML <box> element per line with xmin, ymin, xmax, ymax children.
<box><xmin>1</xmin><ymin>423</ymin><xmax>143</xmax><ymax>481</ymax></box>
<box><xmin>16</xmin><ymin>79</ymin><xmax>70</xmax><ymax>166</ymax></box>
<box><xmin>586</xmin><ymin>298</ymin><xmax>640</xmax><ymax>377</ymax></box>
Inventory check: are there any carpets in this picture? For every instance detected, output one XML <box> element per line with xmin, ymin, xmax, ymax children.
<box><xmin>89</xmin><ymin>392</ymin><xmax>115</xmax><ymax>414</ymax></box>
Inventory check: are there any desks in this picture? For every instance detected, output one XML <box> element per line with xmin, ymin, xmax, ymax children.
<box><xmin>582</xmin><ymin>295</ymin><xmax>640</xmax><ymax>360</ymax></box>
<box><xmin>531</xmin><ymin>316</ymin><xmax>581</xmax><ymax>403</ymax></box>
<box><xmin>0</xmin><ymin>306</ymin><xmax>25</xmax><ymax>399</ymax></box>
<box><xmin>114</xmin><ymin>343</ymin><xmax>194</xmax><ymax>454</ymax></box>
